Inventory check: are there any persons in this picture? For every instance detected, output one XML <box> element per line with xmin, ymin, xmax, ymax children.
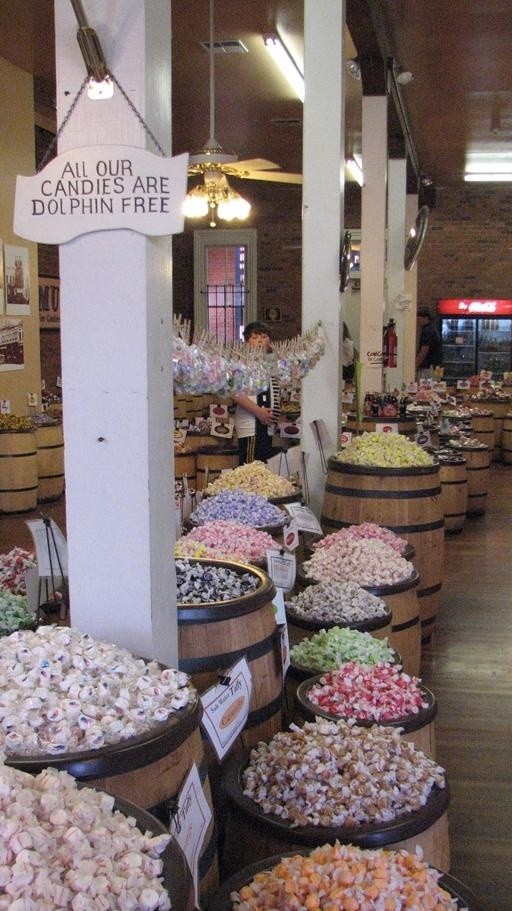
<box><xmin>415</xmin><ymin>309</ymin><xmax>445</xmax><ymax>384</ymax></box>
<box><xmin>231</xmin><ymin>322</ymin><xmax>283</xmax><ymax>466</ymax></box>
<box><xmin>341</xmin><ymin>321</ymin><xmax>357</xmax><ymax>380</ymax></box>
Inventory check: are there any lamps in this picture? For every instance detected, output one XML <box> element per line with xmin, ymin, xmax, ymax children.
<box><xmin>263</xmin><ymin>28</ymin><xmax>364</xmax><ymax>189</ymax></box>
<box><xmin>183</xmin><ymin>170</ymin><xmax>259</xmax><ymax>222</ymax></box>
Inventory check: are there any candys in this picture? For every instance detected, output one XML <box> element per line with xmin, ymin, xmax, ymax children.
<box><xmin>0</xmin><ymin>336</ymin><xmax>492</xmax><ymax>911</ymax></box>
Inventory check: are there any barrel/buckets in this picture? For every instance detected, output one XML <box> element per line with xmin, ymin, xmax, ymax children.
<box><xmin>0</xmin><ymin>427</ymin><xmax>36</xmax><ymax>514</ymax></box>
<box><xmin>2</xmin><ymin>659</ymin><xmax>211</xmax><ymax>860</ymax></box>
<box><xmin>292</xmin><ymin>671</ymin><xmax>441</xmax><ymax>767</ymax></box>
<box><xmin>41</xmin><ymin>777</ymin><xmax>200</xmax><ymax>911</ymax></box>
<box><xmin>225</xmin><ymin>742</ymin><xmax>453</xmax><ymax>877</ymax></box>
<box><xmin>178</xmin><ymin>370</ymin><xmax>511</xmax><ymax>660</ymax></box>
<box><xmin>36</xmin><ymin>422</ymin><xmax>66</xmax><ymax>505</ymax></box>
<box><xmin>173</xmin><ymin>555</ymin><xmax>287</xmax><ymax>749</ymax></box>
<box><xmin>214</xmin><ymin>847</ymin><xmax>481</xmax><ymax>908</ymax></box>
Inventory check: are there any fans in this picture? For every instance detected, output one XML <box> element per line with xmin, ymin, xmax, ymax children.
<box><xmin>183</xmin><ymin>140</ymin><xmax>303</xmax><ymax>224</ymax></box>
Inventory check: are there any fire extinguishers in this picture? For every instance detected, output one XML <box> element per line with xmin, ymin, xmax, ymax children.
<box><xmin>383</xmin><ymin>318</ymin><xmax>397</xmax><ymax>368</ymax></box>
